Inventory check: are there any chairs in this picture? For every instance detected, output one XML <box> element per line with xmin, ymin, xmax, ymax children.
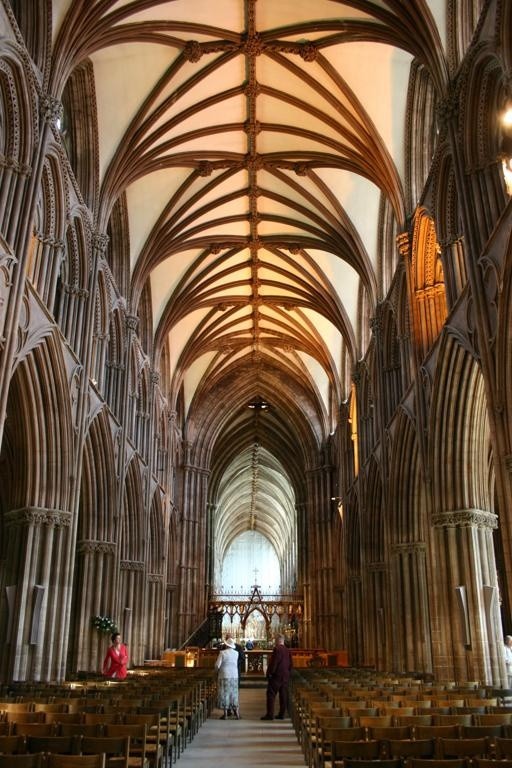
<box><xmin>286</xmin><ymin>665</ymin><xmax>512</xmax><ymax>767</ymax></box>
<box><xmin>0</xmin><ymin>665</ymin><xmax>218</xmax><ymax>768</ymax></box>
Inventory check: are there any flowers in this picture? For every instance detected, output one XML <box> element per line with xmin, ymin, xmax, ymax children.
<box><xmin>92</xmin><ymin>615</ymin><xmax>118</xmax><ymax>636</ymax></box>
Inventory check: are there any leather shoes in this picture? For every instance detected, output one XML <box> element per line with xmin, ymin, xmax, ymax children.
<box><xmin>261</xmin><ymin>714</ymin><xmax>284</xmax><ymax>720</ymax></box>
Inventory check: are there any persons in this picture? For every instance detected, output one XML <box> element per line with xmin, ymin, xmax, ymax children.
<box><xmin>246</xmin><ymin>639</ymin><xmax>255</xmax><ymax>650</ymax></box>
<box><xmin>102</xmin><ymin>632</ymin><xmax>130</xmax><ymax>681</ymax></box>
<box><xmin>289</xmin><ymin>629</ymin><xmax>299</xmax><ymax>648</ymax></box>
<box><xmin>258</xmin><ymin>633</ymin><xmax>290</xmax><ymax>721</ymax></box>
<box><xmin>214</xmin><ymin>637</ymin><xmax>241</xmax><ymax>721</ymax></box>
<box><xmin>220</xmin><ymin>631</ymin><xmax>245</xmax><ymax>690</ymax></box>
<box><xmin>501</xmin><ymin>634</ymin><xmax>512</xmax><ymax>690</ymax></box>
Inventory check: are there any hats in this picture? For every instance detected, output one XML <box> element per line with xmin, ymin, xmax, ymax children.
<box><xmin>224</xmin><ymin>639</ymin><xmax>236</xmax><ymax>649</ymax></box>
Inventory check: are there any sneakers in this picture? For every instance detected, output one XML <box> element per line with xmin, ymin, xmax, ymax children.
<box><xmin>221</xmin><ymin>712</ymin><xmax>241</xmax><ymax>720</ymax></box>
